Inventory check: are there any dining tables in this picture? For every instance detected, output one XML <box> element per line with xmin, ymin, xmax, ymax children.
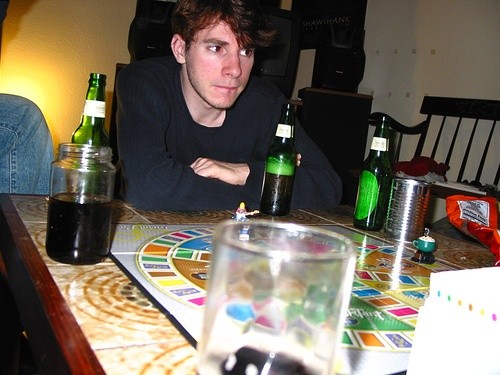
<box><xmin>0</xmin><ymin>192</ymin><xmax>495</xmax><ymax>375</ymax></box>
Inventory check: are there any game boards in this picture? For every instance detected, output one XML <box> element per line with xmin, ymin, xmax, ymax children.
<box><xmin>107</xmin><ymin>223</ymin><xmax>462</xmax><ymax>375</ymax></box>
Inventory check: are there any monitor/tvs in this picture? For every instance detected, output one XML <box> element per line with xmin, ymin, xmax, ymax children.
<box><xmin>250</xmin><ymin>7</ymin><xmax>299</xmax><ymax>97</ymax></box>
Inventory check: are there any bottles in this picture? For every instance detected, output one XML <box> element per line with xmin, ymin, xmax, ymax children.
<box><xmin>260</xmin><ymin>102</ymin><xmax>297</xmax><ymax>217</ymax></box>
<box><xmin>353</xmin><ymin>117</ymin><xmax>393</xmax><ymax>232</ymax></box>
<box><xmin>45</xmin><ymin>143</ymin><xmax>117</xmax><ymax>266</ymax></box>
<box><xmin>71</xmin><ymin>73</ymin><xmax>111</xmax><ymax>144</ymax></box>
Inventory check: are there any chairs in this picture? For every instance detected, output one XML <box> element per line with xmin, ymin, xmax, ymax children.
<box><xmin>368</xmin><ymin>96</ymin><xmax>500</xmax><ymax>186</ymax></box>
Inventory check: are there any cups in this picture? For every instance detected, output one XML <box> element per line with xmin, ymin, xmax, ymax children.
<box><xmin>197</xmin><ymin>220</ymin><xmax>357</xmax><ymax>374</ymax></box>
<box><xmin>412</xmin><ymin>237</ymin><xmax>436</xmax><ymax>252</ymax></box>
<box><xmin>385</xmin><ymin>179</ymin><xmax>430</xmax><ymax>241</ymax></box>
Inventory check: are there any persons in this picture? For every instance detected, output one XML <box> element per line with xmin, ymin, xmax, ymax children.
<box><xmin>0</xmin><ymin>93</ymin><xmax>68</xmax><ymax>195</ymax></box>
<box><xmin>113</xmin><ymin>0</ymin><xmax>343</xmax><ymax>213</ymax></box>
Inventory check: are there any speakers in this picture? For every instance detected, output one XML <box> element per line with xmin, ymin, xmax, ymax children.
<box><xmin>290</xmin><ymin>87</ymin><xmax>373</xmax><ymax>210</ymax></box>
